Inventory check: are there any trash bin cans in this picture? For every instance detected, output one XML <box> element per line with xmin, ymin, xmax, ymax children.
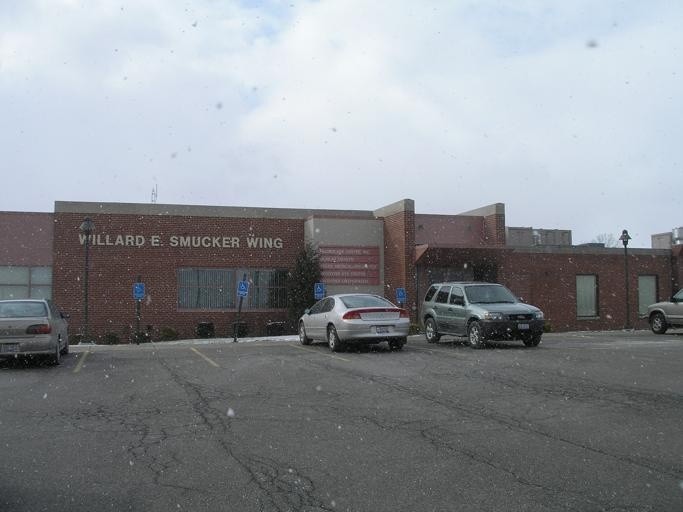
<box><xmin>198</xmin><ymin>322</ymin><xmax>214</xmax><ymax>338</ymax></box>
<box><xmin>233</xmin><ymin>322</ymin><xmax>247</xmax><ymax>338</ymax></box>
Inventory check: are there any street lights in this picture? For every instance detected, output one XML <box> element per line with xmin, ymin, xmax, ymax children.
<box><xmin>617</xmin><ymin>228</ymin><xmax>633</xmax><ymax>330</ymax></box>
<box><xmin>78</xmin><ymin>215</ymin><xmax>95</xmax><ymax>343</ymax></box>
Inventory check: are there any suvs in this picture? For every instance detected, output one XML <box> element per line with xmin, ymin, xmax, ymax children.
<box><xmin>421</xmin><ymin>280</ymin><xmax>546</xmax><ymax>349</ymax></box>
<box><xmin>646</xmin><ymin>287</ymin><xmax>683</xmax><ymax>333</ymax></box>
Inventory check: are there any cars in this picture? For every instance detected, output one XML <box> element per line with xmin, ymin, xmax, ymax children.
<box><xmin>294</xmin><ymin>291</ymin><xmax>412</xmax><ymax>355</ymax></box>
<box><xmin>0</xmin><ymin>297</ymin><xmax>70</xmax><ymax>367</ymax></box>
